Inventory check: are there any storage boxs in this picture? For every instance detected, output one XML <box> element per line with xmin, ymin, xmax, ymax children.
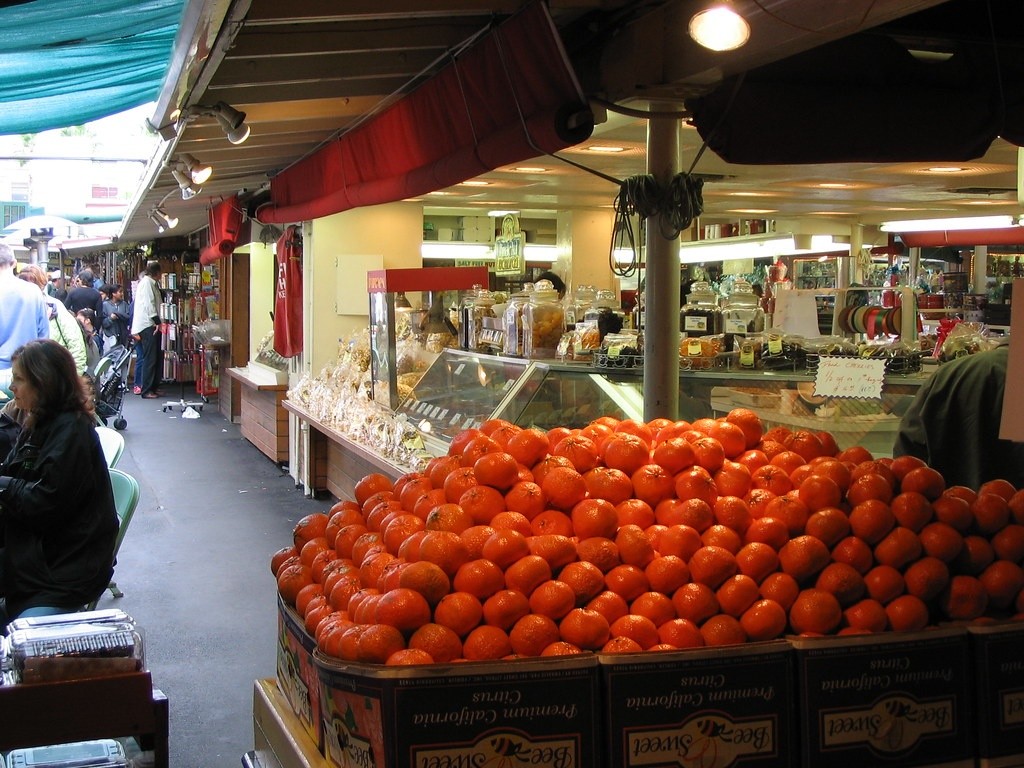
<box><xmin>275</xmin><ymin>590</ymin><xmax>1024</xmax><ymax>768</ymax></box>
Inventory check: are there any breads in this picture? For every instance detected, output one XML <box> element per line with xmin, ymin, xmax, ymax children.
<box><xmin>680</xmin><ymin>337</ymin><xmax>723</xmax><ymax>367</ymax></box>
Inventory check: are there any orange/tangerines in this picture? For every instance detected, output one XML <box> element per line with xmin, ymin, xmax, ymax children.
<box><xmin>271</xmin><ymin>408</ymin><xmax>1024</xmax><ymax>665</ymax></box>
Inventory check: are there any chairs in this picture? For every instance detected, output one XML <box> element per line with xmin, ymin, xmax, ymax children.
<box><xmin>84</xmin><ymin>426</ymin><xmax>141</xmax><ymax>611</ymax></box>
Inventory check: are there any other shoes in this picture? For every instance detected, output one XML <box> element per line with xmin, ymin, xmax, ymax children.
<box><xmin>134</xmin><ymin>385</ymin><xmax>142</xmax><ymax>395</ymax></box>
<box><xmin>144</xmin><ymin>392</ymin><xmax>158</xmax><ymax>398</ymax></box>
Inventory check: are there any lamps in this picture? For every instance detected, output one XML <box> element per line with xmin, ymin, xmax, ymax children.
<box><xmin>147</xmin><ymin>101</ymin><xmax>252</xmax><ymax>233</ymax></box>
<box><xmin>876</xmin><ymin>214</ymin><xmax>1021</xmax><ymax>233</ymax></box>
<box><xmin>421</xmin><ymin>215</ymin><xmax>888</xmax><ymax>264</ymax></box>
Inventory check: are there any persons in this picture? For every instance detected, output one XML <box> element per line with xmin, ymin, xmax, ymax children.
<box><xmin>0</xmin><ymin>243</ymin><xmax>48</xmax><ymax>400</ymax></box>
<box><xmin>20</xmin><ymin>264</ymin><xmax>86</xmax><ymax>377</ymax></box>
<box><xmin>131</xmin><ymin>262</ymin><xmax>166</xmax><ymax>398</ymax></box>
<box><xmin>0</xmin><ymin>340</ymin><xmax>119</xmax><ymax>619</ymax></box>
<box><xmin>894</xmin><ymin>337</ymin><xmax>1024</xmax><ymax>492</ymax></box>
<box><xmin>504</xmin><ymin>271</ymin><xmax>566</xmax><ymax>400</ymax></box>
<box><xmin>50</xmin><ymin>263</ymin><xmax>147</xmax><ymax>395</ymax></box>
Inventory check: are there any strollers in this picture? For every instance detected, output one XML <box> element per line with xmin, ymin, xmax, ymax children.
<box><xmin>90</xmin><ymin>338</ymin><xmax>141</xmax><ymax>430</ymax></box>
